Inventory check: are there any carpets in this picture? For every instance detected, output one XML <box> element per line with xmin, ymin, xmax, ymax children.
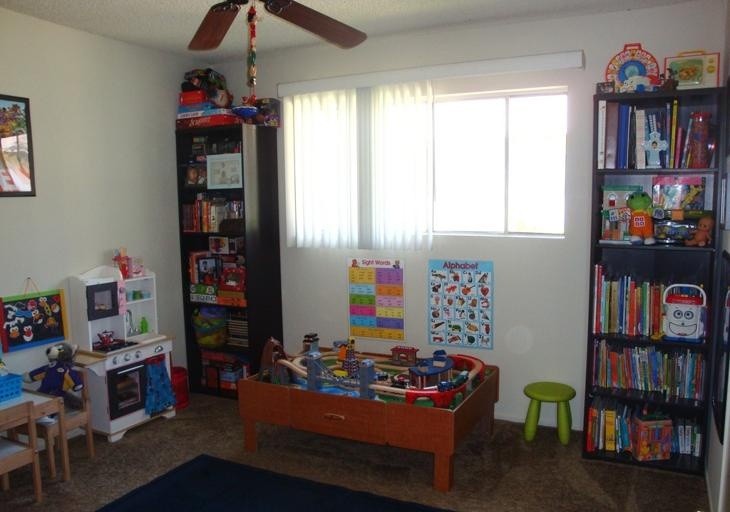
<box><xmin>93</xmin><ymin>453</ymin><xmax>455</xmax><ymax>512</ymax></box>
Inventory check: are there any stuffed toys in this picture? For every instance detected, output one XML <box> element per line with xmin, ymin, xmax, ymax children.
<box><xmin>23</xmin><ymin>343</ymin><xmax>85</xmax><ymax>418</ymax></box>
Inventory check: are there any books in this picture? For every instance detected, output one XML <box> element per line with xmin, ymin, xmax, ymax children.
<box><xmin>185</xmin><ymin>136</ymin><xmax>241</xmax><ymax>163</ymax></box>
<box><xmin>182</xmin><ymin>193</ymin><xmax>241</xmax><ymax>233</ymax></box>
<box><xmin>597</xmin><ymin>99</ymin><xmax>691</xmax><ymax>169</ymax></box>
<box><xmin>587</xmin><ymin>261</ymin><xmax>708</xmax><ymax>458</ymax></box>
<box><xmin>227</xmin><ymin>310</ymin><xmax>249</xmax><ymax>347</ymax></box>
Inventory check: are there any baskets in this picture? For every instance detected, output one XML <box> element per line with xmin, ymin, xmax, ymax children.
<box><xmin>0</xmin><ymin>373</ymin><xmax>23</xmax><ymax>402</ymax></box>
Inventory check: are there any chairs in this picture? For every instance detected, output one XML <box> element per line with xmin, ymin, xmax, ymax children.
<box><xmin>0</xmin><ymin>368</ymin><xmax>96</xmax><ymax>505</ymax></box>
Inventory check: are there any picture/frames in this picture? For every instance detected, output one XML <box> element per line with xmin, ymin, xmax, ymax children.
<box><xmin>0</xmin><ymin>93</ymin><xmax>38</xmax><ymax>197</ymax></box>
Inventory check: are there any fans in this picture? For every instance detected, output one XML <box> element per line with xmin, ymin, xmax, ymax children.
<box><xmin>186</xmin><ymin>0</ymin><xmax>368</xmax><ymax>51</ymax></box>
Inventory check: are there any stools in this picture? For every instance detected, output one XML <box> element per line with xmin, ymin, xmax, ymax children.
<box><xmin>521</xmin><ymin>382</ymin><xmax>577</xmax><ymax>446</ymax></box>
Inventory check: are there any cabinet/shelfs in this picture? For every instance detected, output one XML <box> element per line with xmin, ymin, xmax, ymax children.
<box><xmin>67</xmin><ymin>264</ymin><xmax>178</xmax><ymax>443</ymax></box>
<box><xmin>580</xmin><ymin>86</ymin><xmax>730</xmax><ymax>480</ymax></box>
<box><xmin>173</xmin><ymin>123</ymin><xmax>284</xmax><ymax>400</ymax></box>
